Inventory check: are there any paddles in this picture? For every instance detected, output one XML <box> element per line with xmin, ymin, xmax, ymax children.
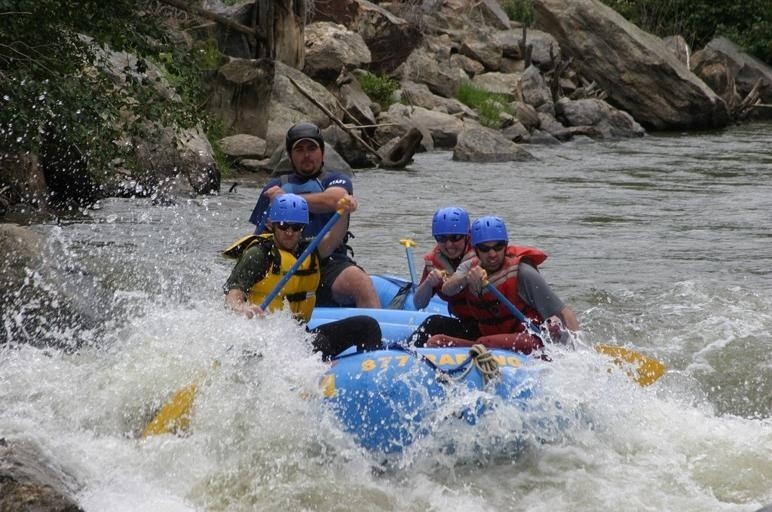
<box><xmin>594</xmin><ymin>347</ymin><xmax>665</xmax><ymax>388</ymax></box>
<box><xmin>140</xmin><ymin>198</ymin><xmax>347</xmax><ymax>437</ymax></box>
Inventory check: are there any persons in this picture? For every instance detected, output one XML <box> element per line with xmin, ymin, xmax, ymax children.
<box><xmin>412</xmin><ymin>205</ymin><xmax>477</xmax><ymax>311</ymax></box>
<box><xmin>402</xmin><ymin>216</ymin><xmax>581</xmax><ymax>355</ymax></box>
<box><xmin>250</xmin><ymin>122</ymin><xmax>381</xmax><ymax>308</ymax></box>
<box><xmin>224</xmin><ymin>193</ymin><xmax>383</xmax><ymax>365</ymax></box>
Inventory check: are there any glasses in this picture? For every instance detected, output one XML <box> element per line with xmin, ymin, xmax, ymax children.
<box><xmin>476</xmin><ymin>241</ymin><xmax>505</xmax><ymax>253</ymax></box>
<box><xmin>274</xmin><ymin>222</ymin><xmax>304</xmax><ymax>232</ymax></box>
<box><xmin>435</xmin><ymin>234</ymin><xmax>464</xmax><ymax>244</ymax></box>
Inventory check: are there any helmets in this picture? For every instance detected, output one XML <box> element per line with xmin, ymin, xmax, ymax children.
<box><xmin>267</xmin><ymin>193</ymin><xmax>311</xmax><ymax>226</ymax></box>
<box><xmin>432</xmin><ymin>207</ymin><xmax>470</xmax><ymax>238</ymax></box>
<box><xmin>286</xmin><ymin>121</ymin><xmax>325</xmax><ymax>155</ymax></box>
<box><xmin>470</xmin><ymin>216</ymin><xmax>509</xmax><ymax>246</ymax></box>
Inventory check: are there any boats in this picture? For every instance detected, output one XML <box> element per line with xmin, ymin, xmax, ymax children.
<box><xmin>307</xmin><ymin>274</ymin><xmax>557</xmax><ymax>478</ymax></box>
